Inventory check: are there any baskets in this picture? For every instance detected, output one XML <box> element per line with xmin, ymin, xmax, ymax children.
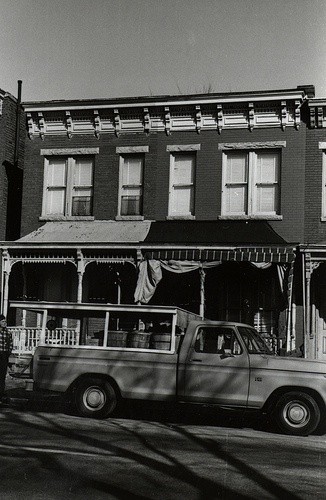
<box><xmin>129</xmin><ymin>331</ymin><xmax>152</xmax><ymax>348</ymax></box>
<box><xmin>153</xmin><ymin>331</ymin><xmax>180</xmax><ymax>351</ymax></box>
<box><xmin>107</xmin><ymin>331</ymin><xmax>128</xmax><ymax>346</ymax></box>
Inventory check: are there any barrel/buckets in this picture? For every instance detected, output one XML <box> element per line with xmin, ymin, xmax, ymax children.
<box><xmin>108</xmin><ymin>330</ymin><xmax>128</xmax><ymax>348</ymax></box>
<box><xmin>131</xmin><ymin>331</ymin><xmax>151</xmax><ymax>348</ymax></box>
<box><xmin>152</xmin><ymin>333</ymin><xmax>179</xmax><ymax>351</ymax></box>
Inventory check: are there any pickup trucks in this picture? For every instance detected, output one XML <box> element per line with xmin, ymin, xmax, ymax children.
<box><xmin>9</xmin><ymin>300</ymin><xmax>326</xmax><ymax>437</ymax></box>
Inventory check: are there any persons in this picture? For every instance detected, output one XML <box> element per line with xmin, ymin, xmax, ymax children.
<box><xmin>0</xmin><ymin>314</ymin><xmax>13</xmax><ymax>390</ymax></box>
